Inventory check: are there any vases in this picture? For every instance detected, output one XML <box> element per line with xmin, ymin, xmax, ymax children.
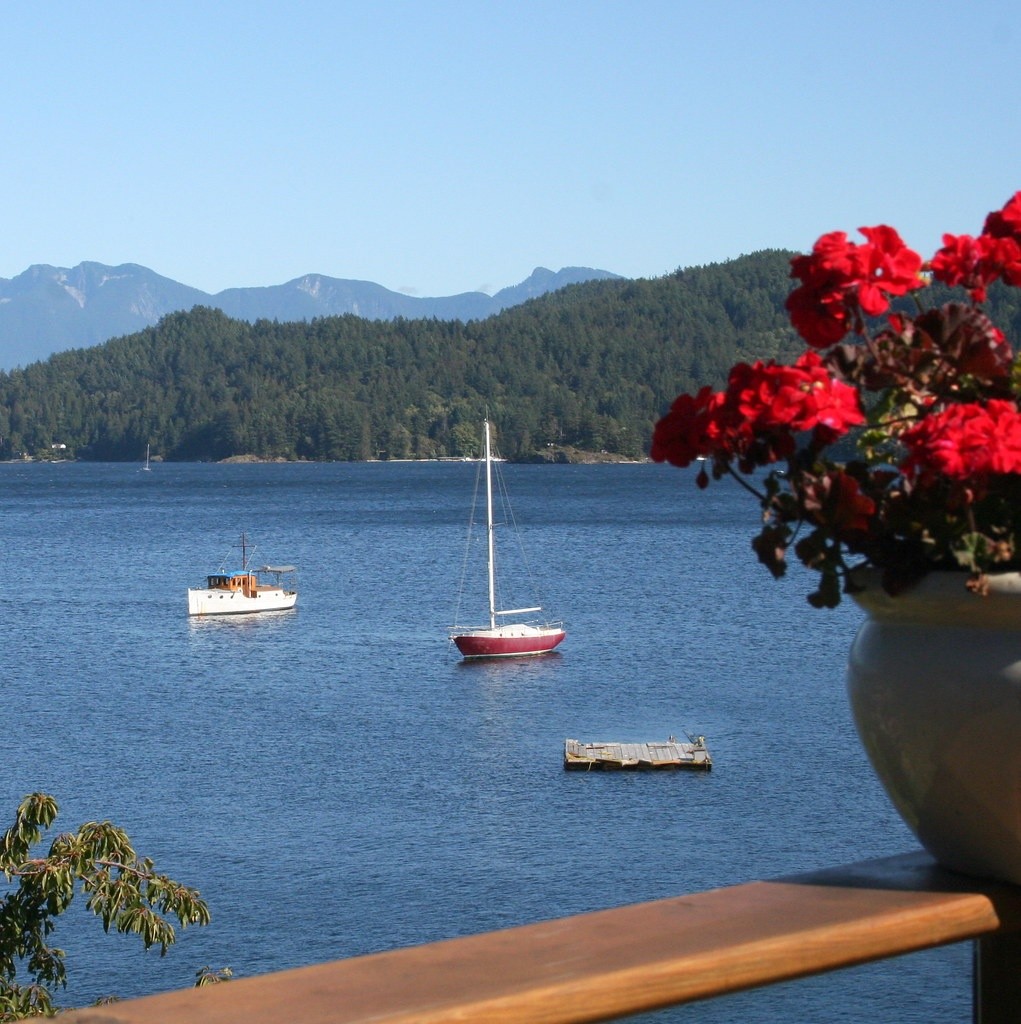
<box><xmin>843</xmin><ymin>570</ymin><xmax>1021</xmax><ymax>884</ymax></box>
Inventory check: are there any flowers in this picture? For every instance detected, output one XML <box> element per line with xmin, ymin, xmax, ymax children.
<box><xmin>647</xmin><ymin>192</ymin><xmax>1021</xmax><ymax>608</ymax></box>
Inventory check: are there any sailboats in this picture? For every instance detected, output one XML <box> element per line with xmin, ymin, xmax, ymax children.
<box><xmin>446</xmin><ymin>416</ymin><xmax>567</xmax><ymax>658</ymax></box>
<box><xmin>142</xmin><ymin>443</ymin><xmax>152</xmax><ymax>470</ymax></box>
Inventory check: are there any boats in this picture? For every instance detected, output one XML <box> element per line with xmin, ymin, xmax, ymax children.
<box><xmin>187</xmin><ymin>531</ymin><xmax>298</xmax><ymax>615</ymax></box>
